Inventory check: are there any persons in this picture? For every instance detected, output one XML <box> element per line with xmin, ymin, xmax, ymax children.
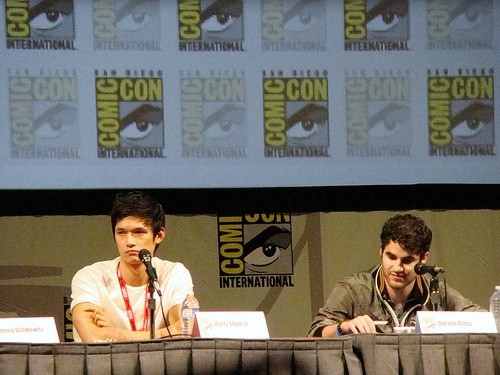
<box><xmin>70</xmin><ymin>191</ymin><xmax>195</xmax><ymax>342</ymax></box>
<box><xmin>306</xmin><ymin>214</ymin><xmax>488</xmax><ymax>337</ymax></box>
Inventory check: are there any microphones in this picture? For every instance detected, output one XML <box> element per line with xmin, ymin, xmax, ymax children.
<box><xmin>139</xmin><ymin>249</ymin><xmax>162</xmax><ymax>296</ymax></box>
<box><xmin>415</xmin><ymin>263</ymin><xmax>442</xmax><ymax>275</ymax></box>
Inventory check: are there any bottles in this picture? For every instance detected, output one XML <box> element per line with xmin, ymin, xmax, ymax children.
<box><xmin>490</xmin><ymin>286</ymin><xmax>500</xmax><ymax>333</ymax></box>
<box><xmin>182</xmin><ymin>291</ymin><xmax>200</xmax><ymax>338</ymax></box>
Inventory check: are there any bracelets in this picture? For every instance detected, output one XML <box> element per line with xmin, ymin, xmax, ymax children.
<box><xmin>336</xmin><ymin>322</ymin><xmax>345</xmax><ymax>336</ymax></box>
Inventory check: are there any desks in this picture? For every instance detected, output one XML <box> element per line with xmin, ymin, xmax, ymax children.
<box><xmin>0</xmin><ymin>333</ymin><xmax>500</xmax><ymax>375</ymax></box>
